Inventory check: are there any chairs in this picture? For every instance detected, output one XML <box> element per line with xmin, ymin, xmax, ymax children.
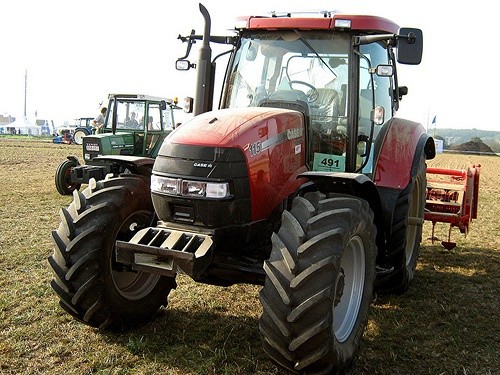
<box><xmin>303</xmin><ymin>88</ymin><xmax>339</xmax><ymax>130</ymax></box>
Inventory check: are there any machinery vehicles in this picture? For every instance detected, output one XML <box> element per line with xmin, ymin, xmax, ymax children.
<box><xmin>47</xmin><ymin>3</ymin><xmax>480</xmax><ymax>374</ymax></box>
<box><xmin>53</xmin><ymin>116</ymin><xmax>93</xmax><ymax>144</ymax></box>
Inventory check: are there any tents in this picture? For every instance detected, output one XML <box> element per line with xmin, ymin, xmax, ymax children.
<box><xmin>3</xmin><ymin>118</ymin><xmax>41</xmax><ymax>137</ymax></box>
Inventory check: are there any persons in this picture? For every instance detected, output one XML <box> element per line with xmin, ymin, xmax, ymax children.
<box><xmin>124</xmin><ymin>112</ymin><xmax>138</xmax><ymax>129</ymax></box>
<box><xmin>92</xmin><ymin>107</ymin><xmax>107</xmax><ymax>135</ymax></box>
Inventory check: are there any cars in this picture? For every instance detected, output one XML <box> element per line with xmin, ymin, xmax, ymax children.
<box><xmin>55</xmin><ymin>93</ymin><xmax>186</xmax><ymax>195</ymax></box>
